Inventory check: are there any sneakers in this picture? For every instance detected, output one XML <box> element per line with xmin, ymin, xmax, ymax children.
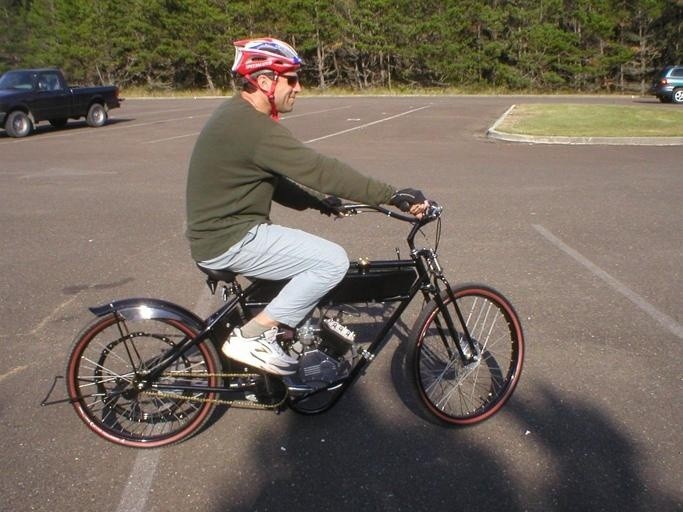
<box><xmin>222</xmin><ymin>325</ymin><xmax>300</xmax><ymax>403</ymax></box>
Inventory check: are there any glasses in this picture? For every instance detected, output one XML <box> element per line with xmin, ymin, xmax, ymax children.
<box><xmin>280</xmin><ymin>74</ymin><xmax>297</xmax><ymax>89</ymax></box>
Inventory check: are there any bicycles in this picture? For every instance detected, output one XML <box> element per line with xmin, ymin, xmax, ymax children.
<box><xmin>39</xmin><ymin>190</ymin><xmax>526</xmax><ymax>449</ymax></box>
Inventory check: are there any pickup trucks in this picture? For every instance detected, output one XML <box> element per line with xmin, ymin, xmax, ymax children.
<box><xmin>0</xmin><ymin>68</ymin><xmax>123</xmax><ymax>138</ymax></box>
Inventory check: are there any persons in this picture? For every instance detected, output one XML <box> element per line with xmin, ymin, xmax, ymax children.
<box><xmin>184</xmin><ymin>36</ymin><xmax>431</xmax><ymax>376</ymax></box>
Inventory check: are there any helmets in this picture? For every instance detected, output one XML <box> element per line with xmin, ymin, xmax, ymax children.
<box><xmin>231</xmin><ymin>38</ymin><xmax>309</xmax><ymax>76</ymax></box>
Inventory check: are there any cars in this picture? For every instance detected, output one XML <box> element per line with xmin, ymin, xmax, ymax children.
<box><xmin>650</xmin><ymin>64</ymin><xmax>682</xmax><ymax>104</ymax></box>
